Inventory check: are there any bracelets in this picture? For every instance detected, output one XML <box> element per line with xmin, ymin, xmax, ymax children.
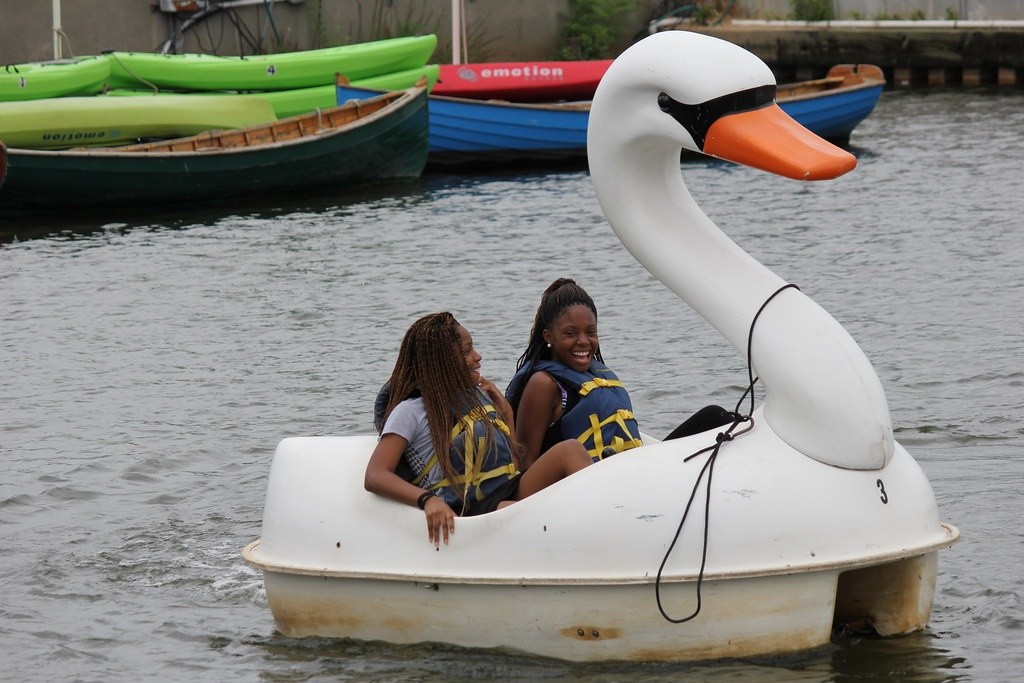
<box><xmin>417</xmin><ymin>490</ymin><xmax>436</xmax><ymax>510</ymax></box>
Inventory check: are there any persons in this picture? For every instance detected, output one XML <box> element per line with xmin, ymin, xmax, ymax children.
<box><xmin>364</xmin><ymin>312</ymin><xmax>594</xmax><ymax>547</ymax></box>
<box><xmin>503</xmin><ymin>279</ymin><xmax>736</xmax><ymax>463</ymax></box>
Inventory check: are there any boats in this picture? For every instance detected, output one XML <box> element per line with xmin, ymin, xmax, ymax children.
<box><xmin>329</xmin><ymin>64</ymin><xmax>887</xmax><ymax>171</ymax></box>
<box><xmin>0</xmin><ymin>35</ymin><xmax>619</xmax><ymax>152</ymax></box>
<box><xmin>1</xmin><ymin>77</ymin><xmax>431</xmax><ymax>213</ymax></box>
<box><xmin>239</xmin><ymin>28</ymin><xmax>959</xmax><ymax>666</ymax></box>
